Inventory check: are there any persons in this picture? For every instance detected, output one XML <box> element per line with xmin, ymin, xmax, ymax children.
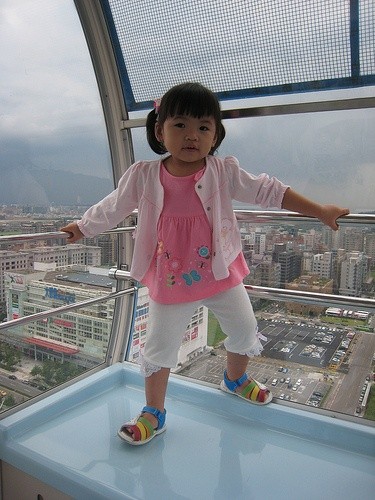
<box><xmin>59</xmin><ymin>81</ymin><xmax>349</xmax><ymax>446</ymax></box>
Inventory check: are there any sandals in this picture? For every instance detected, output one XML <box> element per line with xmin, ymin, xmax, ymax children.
<box><xmin>219</xmin><ymin>369</ymin><xmax>273</xmax><ymax>405</ymax></box>
<box><xmin>118</xmin><ymin>406</ymin><xmax>167</xmax><ymax>446</ymax></box>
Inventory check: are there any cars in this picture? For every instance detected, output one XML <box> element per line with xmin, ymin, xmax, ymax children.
<box><xmin>211</xmin><ymin>302</ymin><xmax>370</xmax><ymax>414</ymax></box>
